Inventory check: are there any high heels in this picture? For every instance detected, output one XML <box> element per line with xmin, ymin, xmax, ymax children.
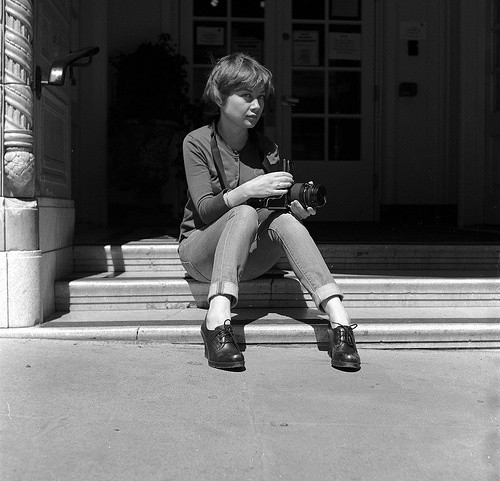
<box><xmin>200</xmin><ymin>310</ymin><xmax>245</xmax><ymax>369</ymax></box>
<box><xmin>327</xmin><ymin>322</ymin><xmax>360</xmax><ymax>369</ymax></box>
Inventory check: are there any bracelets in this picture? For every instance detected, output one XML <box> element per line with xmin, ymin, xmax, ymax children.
<box><xmin>227</xmin><ymin>188</ymin><xmax>232</xmax><ymax>208</ymax></box>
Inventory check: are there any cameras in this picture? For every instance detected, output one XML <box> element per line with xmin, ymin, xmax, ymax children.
<box><xmin>265</xmin><ymin>183</ymin><xmax>327</xmax><ymax>210</ymax></box>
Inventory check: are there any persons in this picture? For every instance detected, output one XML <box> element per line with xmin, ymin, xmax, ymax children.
<box><xmin>177</xmin><ymin>51</ymin><xmax>360</xmax><ymax>370</ymax></box>
